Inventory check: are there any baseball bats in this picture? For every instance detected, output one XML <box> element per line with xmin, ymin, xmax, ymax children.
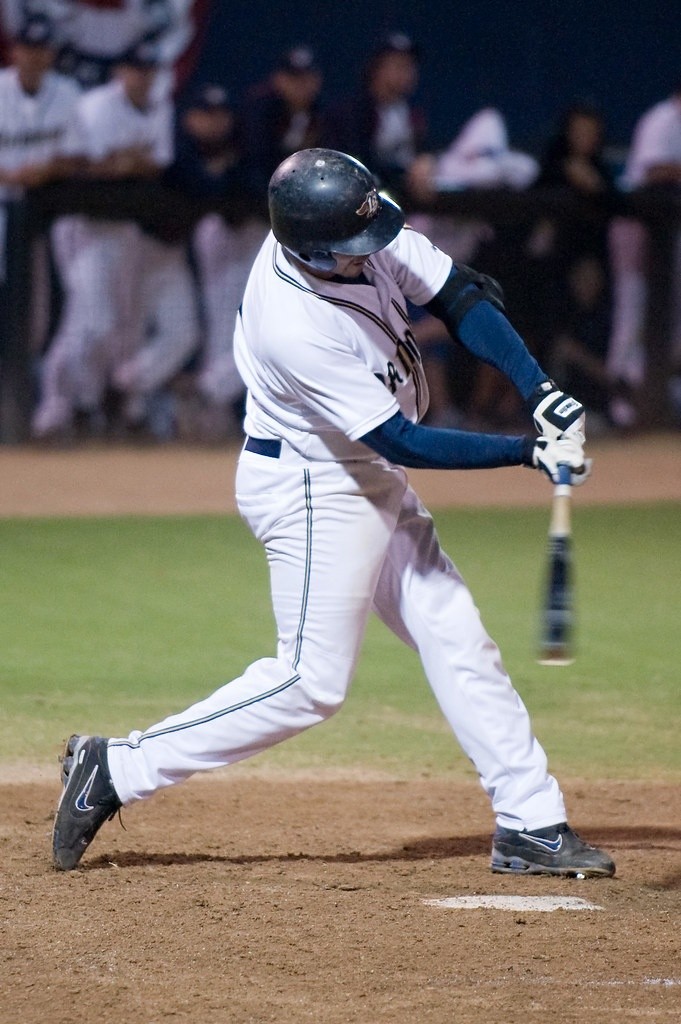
<box><xmin>531</xmin><ymin>465</ymin><xmax>577</xmax><ymax>670</ymax></box>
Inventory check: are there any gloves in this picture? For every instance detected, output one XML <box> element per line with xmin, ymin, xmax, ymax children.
<box><xmin>532</xmin><ymin>436</ymin><xmax>593</xmax><ymax>486</ymax></box>
<box><xmin>525</xmin><ymin>379</ymin><xmax>585</xmax><ymax>446</ymax></box>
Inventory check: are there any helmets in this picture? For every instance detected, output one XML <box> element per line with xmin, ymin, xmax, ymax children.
<box><xmin>269</xmin><ymin>147</ymin><xmax>405</xmax><ymax>274</ymax></box>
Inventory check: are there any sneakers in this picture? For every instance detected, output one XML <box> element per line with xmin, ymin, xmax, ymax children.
<box><xmin>51</xmin><ymin>734</ymin><xmax>123</xmax><ymax>872</ymax></box>
<box><xmin>491</xmin><ymin>821</ymin><xmax>616</xmax><ymax>880</ymax></box>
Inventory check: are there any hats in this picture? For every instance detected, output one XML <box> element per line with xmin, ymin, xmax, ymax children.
<box><xmin>14</xmin><ymin>16</ymin><xmax>56</xmax><ymax>49</ymax></box>
<box><xmin>277</xmin><ymin>50</ymin><xmax>319</xmax><ymax>79</ymax></box>
<box><xmin>121</xmin><ymin>46</ymin><xmax>161</xmax><ymax>69</ymax></box>
<box><xmin>377</xmin><ymin>31</ymin><xmax>415</xmax><ymax>50</ymax></box>
<box><xmin>192</xmin><ymin>90</ymin><xmax>231</xmax><ymax>112</ymax></box>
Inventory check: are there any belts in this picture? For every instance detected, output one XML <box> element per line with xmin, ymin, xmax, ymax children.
<box><xmin>245</xmin><ymin>435</ymin><xmax>282</xmax><ymax>459</ymax></box>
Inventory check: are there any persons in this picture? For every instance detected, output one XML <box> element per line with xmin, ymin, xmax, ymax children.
<box><xmin>52</xmin><ymin>149</ymin><xmax>615</xmax><ymax>879</ymax></box>
<box><xmin>0</xmin><ymin>0</ymin><xmax>681</xmax><ymax>436</ymax></box>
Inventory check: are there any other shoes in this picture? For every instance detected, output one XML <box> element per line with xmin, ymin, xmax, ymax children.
<box><xmin>32</xmin><ymin>392</ymin><xmax>242</xmax><ymax>446</ymax></box>
<box><xmin>601</xmin><ymin>384</ymin><xmax>644</xmax><ymax>429</ymax></box>
